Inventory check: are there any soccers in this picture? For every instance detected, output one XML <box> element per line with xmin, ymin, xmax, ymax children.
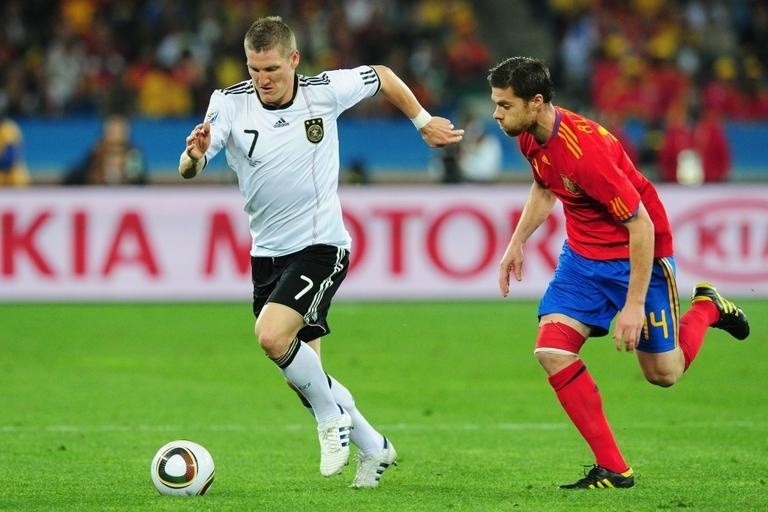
<box><xmin>151</xmin><ymin>439</ymin><xmax>216</xmax><ymax>495</ymax></box>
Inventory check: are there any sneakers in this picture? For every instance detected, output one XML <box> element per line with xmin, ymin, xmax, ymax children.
<box><xmin>349</xmin><ymin>436</ymin><xmax>398</xmax><ymax>488</ymax></box>
<box><xmin>314</xmin><ymin>401</ymin><xmax>354</xmax><ymax>477</ymax></box>
<box><xmin>557</xmin><ymin>464</ymin><xmax>634</xmax><ymax>490</ymax></box>
<box><xmin>691</xmin><ymin>283</ymin><xmax>750</xmax><ymax>340</ymax></box>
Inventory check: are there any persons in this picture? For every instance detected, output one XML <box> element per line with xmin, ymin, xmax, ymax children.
<box><xmin>179</xmin><ymin>15</ymin><xmax>465</xmax><ymax>489</ymax></box>
<box><xmin>488</xmin><ymin>57</ymin><xmax>749</xmax><ymax>489</ymax></box>
